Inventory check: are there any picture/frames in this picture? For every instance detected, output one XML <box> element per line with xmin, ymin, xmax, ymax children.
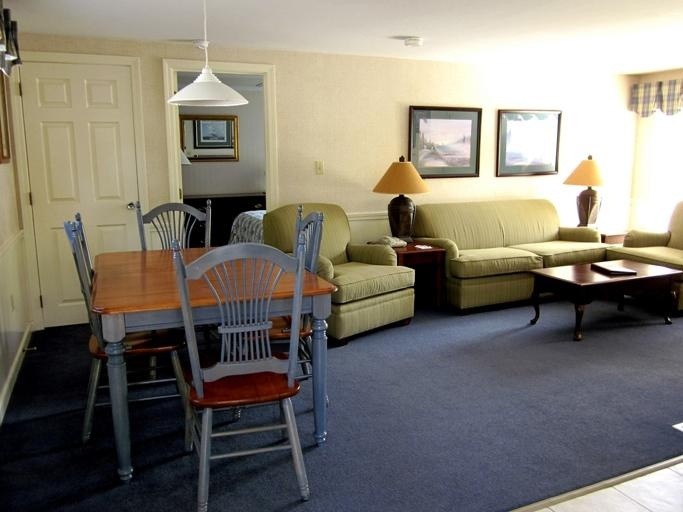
<box><xmin>193</xmin><ymin>120</ymin><xmax>234</xmax><ymax>148</ymax></box>
<box><xmin>495</xmin><ymin>109</ymin><xmax>562</xmax><ymax>178</ymax></box>
<box><xmin>178</xmin><ymin>115</ymin><xmax>239</xmax><ymax>162</ymax></box>
<box><xmin>408</xmin><ymin>105</ymin><xmax>483</xmax><ymax>179</ymax></box>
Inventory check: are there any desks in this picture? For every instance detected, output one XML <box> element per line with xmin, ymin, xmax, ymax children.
<box><xmin>93</xmin><ymin>244</ymin><xmax>337</xmax><ymax>482</ymax></box>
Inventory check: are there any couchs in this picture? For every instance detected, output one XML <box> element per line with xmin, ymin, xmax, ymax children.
<box><xmin>415</xmin><ymin>198</ymin><xmax>614</xmax><ymax>310</ymax></box>
<box><xmin>263</xmin><ymin>203</ymin><xmax>416</xmax><ymax>340</ymax></box>
<box><xmin>606</xmin><ymin>202</ymin><xmax>683</xmax><ymax>314</ymax></box>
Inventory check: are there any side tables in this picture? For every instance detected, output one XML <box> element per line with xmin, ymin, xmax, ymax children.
<box><xmin>367</xmin><ymin>238</ymin><xmax>447</xmax><ymax>326</ymax></box>
<box><xmin>599</xmin><ymin>233</ymin><xmax>627</xmax><ymax>244</ymax></box>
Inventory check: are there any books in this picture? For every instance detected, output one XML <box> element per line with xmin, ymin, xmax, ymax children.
<box><xmin>591</xmin><ymin>261</ymin><xmax>637</xmax><ymax>277</ymax></box>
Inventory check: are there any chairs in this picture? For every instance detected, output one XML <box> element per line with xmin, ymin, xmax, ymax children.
<box><xmin>61</xmin><ymin>213</ymin><xmax>199</xmax><ymax>442</ymax></box>
<box><xmin>168</xmin><ymin>233</ymin><xmax>321</xmax><ymax>512</ymax></box>
<box><xmin>134</xmin><ymin>199</ymin><xmax>213</xmax><ymax>252</ymax></box>
<box><xmin>262</xmin><ymin>205</ymin><xmax>330</xmax><ymax>407</ymax></box>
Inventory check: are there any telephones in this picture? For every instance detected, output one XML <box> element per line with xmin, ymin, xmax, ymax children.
<box><xmin>378</xmin><ymin>235</ymin><xmax>407</xmax><ymax>246</ymax></box>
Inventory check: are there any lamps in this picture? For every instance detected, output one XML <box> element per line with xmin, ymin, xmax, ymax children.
<box><xmin>167</xmin><ymin>0</ymin><xmax>249</xmax><ymax>107</ymax></box>
<box><xmin>371</xmin><ymin>155</ymin><xmax>428</xmax><ymax>243</ymax></box>
<box><xmin>563</xmin><ymin>155</ymin><xmax>604</xmax><ymax>227</ymax></box>
